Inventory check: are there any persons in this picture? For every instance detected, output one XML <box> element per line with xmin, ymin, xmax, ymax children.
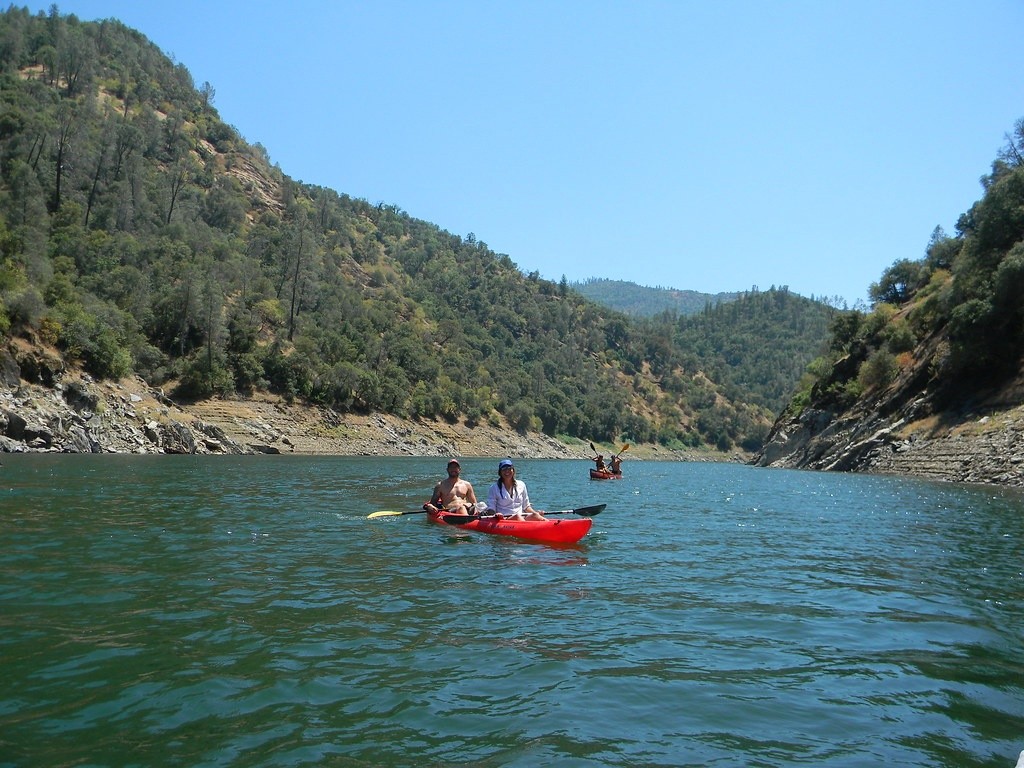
<box><xmin>422</xmin><ymin>459</ymin><xmax>478</xmax><ymax>515</ymax></box>
<box><xmin>486</xmin><ymin>459</ymin><xmax>549</xmax><ymax>521</ymax></box>
<box><xmin>591</xmin><ymin>454</ymin><xmax>622</xmax><ymax>474</ymax></box>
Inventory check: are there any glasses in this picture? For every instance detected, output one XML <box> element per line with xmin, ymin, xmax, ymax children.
<box><xmin>501</xmin><ymin>466</ymin><xmax>512</xmax><ymax>471</ymax></box>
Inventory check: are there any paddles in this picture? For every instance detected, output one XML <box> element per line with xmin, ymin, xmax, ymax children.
<box><xmin>366</xmin><ymin>507</ymin><xmax>451</xmax><ymax>520</ymax></box>
<box><xmin>443</xmin><ymin>503</ymin><xmax>606</xmax><ymax>526</ymax></box>
<box><xmin>590</xmin><ymin>442</ymin><xmax>610</xmax><ymax>479</ymax></box>
<box><xmin>606</xmin><ymin>444</ymin><xmax>629</xmax><ymax>467</ymax></box>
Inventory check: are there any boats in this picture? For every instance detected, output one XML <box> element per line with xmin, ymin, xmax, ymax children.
<box><xmin>589</xmin><ymin>468</ymin><xmax>622</xmax><ymax>479</ymax></box>
<box><xmin>424</xmin><ymin>499</ymin><xmax>593</xmax><ymax>542</ymax></box>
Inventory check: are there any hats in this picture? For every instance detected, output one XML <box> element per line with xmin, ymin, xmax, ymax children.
<box><xmin>448</xmin><ymin>459</ymin><xmax>460</xmax><ymax>467</ymax></box>
<box><xmin>499</xmin><ymin>459</ymin><xmax>513</xmax><ymax>468</ymax></box>
